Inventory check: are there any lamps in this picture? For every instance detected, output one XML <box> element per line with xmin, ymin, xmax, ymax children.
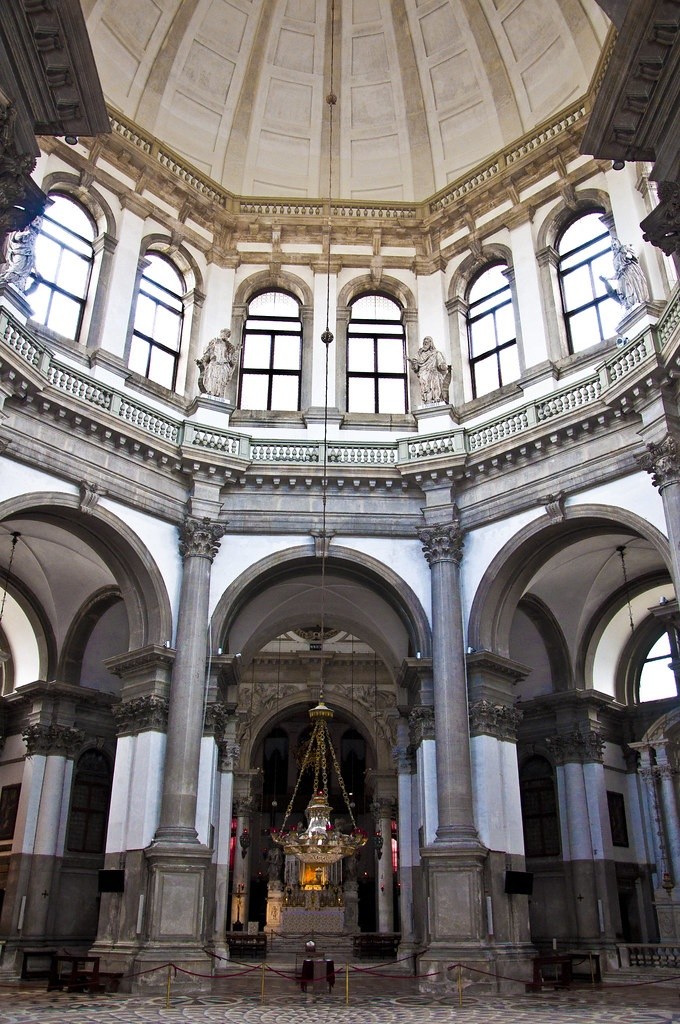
<box><xmin>273</xmin><ymin>764</ymin><xmax>278</xmax><ymax>807</ymax></box>
<box><xmin>269</xmin><ymin>0</ymin><xmax>368</xmax><ymax>893</ymax></box>
<box><xmin>238</xmin><ymin>828</ymin><xmax>251</xmax><ymax>858</ymax></box>
<box><xmin>373</xmin><ymin>830</ymin><xmax>384</xmax><ymax>860</ymax></box>
<box><xmin>380</xmin><ymin>885</ymin><xmax>384</xmax><ymax>892</ymax></box>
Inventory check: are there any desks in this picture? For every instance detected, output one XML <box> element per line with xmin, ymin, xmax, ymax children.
<box><xmin>538</xmin><ymin>949</ymin><xmax>603</xmax><ymax>992</ymax></box>
<box><xmin>47</xmin><ymin>956</ymin><xmax>101</xmax><ymax>993</ymax></box>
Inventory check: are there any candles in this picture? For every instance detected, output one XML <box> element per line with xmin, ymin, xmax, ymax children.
<box><xmin>552</xmin><ymin>938</ymin><xmax>556</xmax><ymax>949</ymax></box>
